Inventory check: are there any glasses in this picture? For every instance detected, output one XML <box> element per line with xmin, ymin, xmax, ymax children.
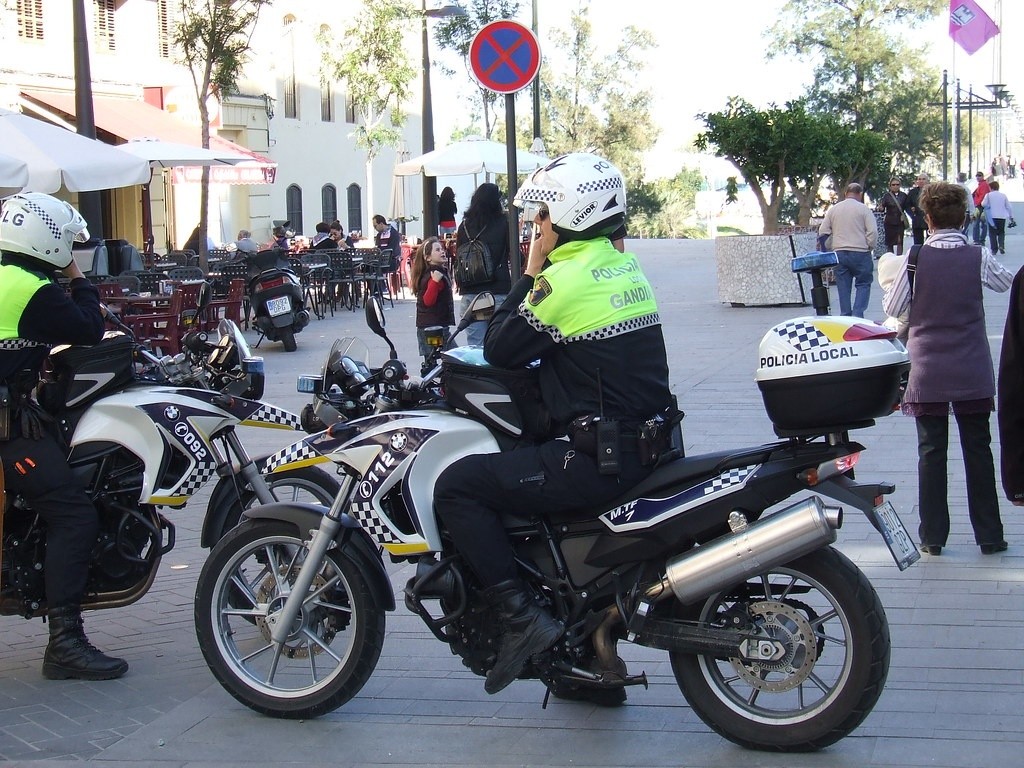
<box><xmin>892</xmin><ymin>184</ymin><xmax>900</xmax><ymax>186</ymax></box>
<box><xmin>976</xmin><ymin>175</ymin><xmax>981</xmax><ymax>177</ymax></box>
<box><xmin>539</xmin><ymin>208</ymin><xmax>549</xmax><ymax>219</ymax></box>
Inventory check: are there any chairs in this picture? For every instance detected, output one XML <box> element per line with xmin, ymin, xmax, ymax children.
<box><xmin>63</xmin><ymin>244</ymin><xmax>415</xmax><ymax>357</ymax></box>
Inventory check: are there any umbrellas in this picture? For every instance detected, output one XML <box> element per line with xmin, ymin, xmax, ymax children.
<box><xmin>394</xmin><ymin>135</ymin><xmax>554</xmax><ymax>177</ymax></box>
<box><xmin>114</xmin><ymin>140</ymin><xmax>255</xmax><ymax>276</ymax></box>
<box><xmin>0</xmin><ymin>107</ymin><xmax>151</xmax><ymax>194</ymax></box>
<box><xmin>387</xmin><ymin>139</ymin><xmax>420</xmax><ymax>234</ymax></box>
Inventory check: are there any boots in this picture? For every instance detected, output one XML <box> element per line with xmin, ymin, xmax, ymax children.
<box><xmin>484</xmin><ymin>579</ymin><xmax>566</xmax><ymax>695</ymax></box>
<box><xmin>43</xmin><ymin>608</ymin><xmax>129</xmax><ymax>680</ymax></box>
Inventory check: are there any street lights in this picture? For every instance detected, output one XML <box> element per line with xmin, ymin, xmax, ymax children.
<box><xmin>926</xmin><ymin>70</ymin><xmax>1024</xmax><ymax>184</ymax></box>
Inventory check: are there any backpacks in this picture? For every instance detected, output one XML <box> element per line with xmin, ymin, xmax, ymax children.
<box><xmin>454</xmin><ymin>221</ymin><xmax>506</xmax><ymax>288</ymax></box>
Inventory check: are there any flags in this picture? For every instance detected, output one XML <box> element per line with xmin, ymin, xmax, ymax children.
<box><xmin>950</xmin><ymin>1</ymin><xmax>1002</xmax><ymax>56</ymax></box>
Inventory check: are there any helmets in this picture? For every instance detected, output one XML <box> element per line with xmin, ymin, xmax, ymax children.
<box><xmin>0</xmin><ymin>192</ymin><xmax>91</xmax><ymax>269</ymax></box>
<box><xmin>514</xmin><ymin>153</ymin><xmax>626</xmax><ymax>239</ymax></box>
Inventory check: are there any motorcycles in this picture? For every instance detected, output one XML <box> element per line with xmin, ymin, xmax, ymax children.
<box><xmin>194</xmin><ymin>251</ymin><xmax>921</xmax><ymax>754</ymax></box>
<box><xmin>225</xmin><ymin>228</ymin><xmax>311</xmax><ymax>352</ymax></box>
<box><xmin>0</xmin><ymin>281</ymin><xmax>341</xmax><ymax>720</ymax></box>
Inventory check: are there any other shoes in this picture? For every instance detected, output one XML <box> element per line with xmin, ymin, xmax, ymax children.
<box><xmin>919</xmin><ymin>542</ymin><xmax>941</xmax><ymax>555</ymax></box>
<box><xmin>973</xmin><ymin>241</ymin><xmax>985</xmax><ymax>246</ymax></box>
<box><xmin>992</xmin><ymin>247</ymin><xmax>1005</xmax><ymax>255</ymax></box>
<box><xmin>981</xmin><ymin>541</ymin><xmax>1007</xmax><ymax>554</ymax></box>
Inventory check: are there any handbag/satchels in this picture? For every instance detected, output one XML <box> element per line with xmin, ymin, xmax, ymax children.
<box><xmin>902</xmin><ymin>213</ymin><xmax>909</xmax><ymax>229</ymax></box>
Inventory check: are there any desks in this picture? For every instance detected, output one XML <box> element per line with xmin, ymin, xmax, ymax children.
<box><xmin>106</xmin><ymin>296</ymin><xmax>170</xmax><ymax>303</ymax></box>
<box><xmin>293</xmin><ymin>263</ymin><xmax>327</xmax><ymax>320</ymax></box>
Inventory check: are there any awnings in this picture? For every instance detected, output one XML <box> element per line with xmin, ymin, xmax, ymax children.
<box><xmin>19</xmin><ymin>89</ymin><xmax>278</xmax><ymax>185</ymax></box>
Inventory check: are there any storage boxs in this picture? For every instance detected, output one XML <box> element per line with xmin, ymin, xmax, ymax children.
<box><xmin>754</xmin><ymin>316</ymin><xmax>911</xmax><ymax>430</ymax></box>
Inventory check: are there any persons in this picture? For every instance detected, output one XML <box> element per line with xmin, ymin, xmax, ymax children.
<box><xmin>409</xmin><ymin>182</ymin><xmax>525</xmax><ymax>380</ymax></box>
<box><xmin>817</xmin><ymin>180</ymin><xmax>1024</xmax><ymax>556</ymax></box>
<box><xmin>432</xmin><ymin>153</ymin><xmax>671</xmax><ymax>708</ymax></box>
<box><xmin>183</xmin><ymin>215</ymin><xmax>403</xmax><ymax>304</ymax></box>
<box><xmin>878</xmin><ymin>171</ymin><xmax>1014</xmax><ymax>256</ymax></box>
<box><xmin>0</xmin><ymin>192</ymin><xmax>128</xmax><ymax>682</ymax></box>
<box><xmin>991</xmin><ymin>152</ymin><xmax>1017</xmax><ymax>185</ymax></box>
<box><xmin>436</xmin><ymin>187</ymin><xmax>458</xmax><ymax>235</ymax></box>
